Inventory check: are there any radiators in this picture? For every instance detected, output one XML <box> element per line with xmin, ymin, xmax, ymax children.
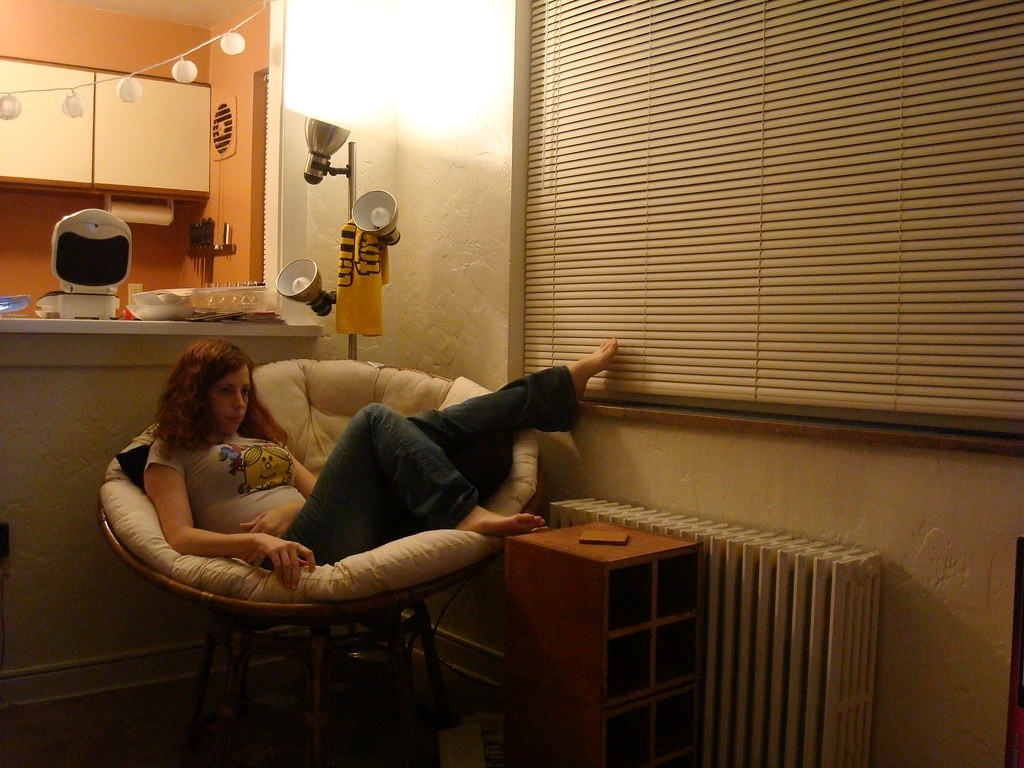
<box><xmin>551</xmin><ymin>497</ymin><xmax>881</xmax><ymax>767</ymax></box>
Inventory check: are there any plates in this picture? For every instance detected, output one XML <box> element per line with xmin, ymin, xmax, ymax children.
<box><xmin>35</xmin><ymin>310</ymin><xmax>61</xmax><ymax>319</ymax></box>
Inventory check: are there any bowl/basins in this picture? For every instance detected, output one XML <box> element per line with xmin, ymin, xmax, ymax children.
<box><xmin>126</xmin><ymin>305</ymin><xmax>197</xmax><ymax>321</ymax></box>
<box><xmin>0</xmin><ymin>294</ymin><xmax>31</xmax><ymax>313</ymax></box>
<box><xmin>133</xmin><ymin>288</ymin><xmax>195</xmax><ymax>305</ymax></box>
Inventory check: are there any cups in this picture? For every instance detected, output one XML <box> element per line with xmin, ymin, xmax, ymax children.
<box><xmin>203</xmin><ymin>281</ymin><xmax>257</xmax><ymax>313</ymax></box>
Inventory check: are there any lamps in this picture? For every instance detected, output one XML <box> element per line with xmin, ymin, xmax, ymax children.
<box><xmin>275</xmin><ymin>119</ymin><xmax>400</xmax><ymax>362</ymax></box>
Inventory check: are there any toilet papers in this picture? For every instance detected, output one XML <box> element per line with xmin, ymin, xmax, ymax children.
<box><xmin>111</xmin><ymin>202</ymin><xmax>172</xmax><ymax>226</ymax></box>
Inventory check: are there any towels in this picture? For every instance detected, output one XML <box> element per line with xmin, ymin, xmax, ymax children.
<box><xmin>336</xmin><ymin>217</ymin><xmax>389</xmax><ymax>337</ymax></box>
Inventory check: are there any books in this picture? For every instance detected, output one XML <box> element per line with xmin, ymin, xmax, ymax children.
<box><xmin>438</xmin><ymin>708</ymin><xmax>505</xmax><ymax>768</ymax></box>
<box><xmin>133</xmin><ymin>285</ymin><xmax>286</xmax><ymax>324</ymax></box>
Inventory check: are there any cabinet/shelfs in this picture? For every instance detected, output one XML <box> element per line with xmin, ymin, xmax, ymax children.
<box><xmin>0</xmin><ymin>56</ymin><xmax>212</xmax><ymax>207</ymax></box>
<box><xmin>506</xmin><ymin>523</ymin><xmax>706</xmax><ymax>767</ymax></box>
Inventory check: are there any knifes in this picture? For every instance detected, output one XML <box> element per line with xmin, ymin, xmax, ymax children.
<box><xmin>223</xmin><ymin>223</ymin><xmax>229</xmax><ymax>258</ymax></box>
<box><xmin>190</xmin><ymin>218</ymin><xmax>214</xmax><ymax>287</ymax></box>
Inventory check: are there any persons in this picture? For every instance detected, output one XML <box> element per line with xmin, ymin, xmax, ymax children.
<box><xmin>142</xmin><ymin>338</ymin><xmax>619</xmax><ymax>590</ymax></box>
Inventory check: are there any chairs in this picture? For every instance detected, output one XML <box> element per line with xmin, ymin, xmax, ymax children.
<box><xmin>96</xmin><ymin>359</ymin><xmax>545</xmax><ymax>768</ymax></box>
